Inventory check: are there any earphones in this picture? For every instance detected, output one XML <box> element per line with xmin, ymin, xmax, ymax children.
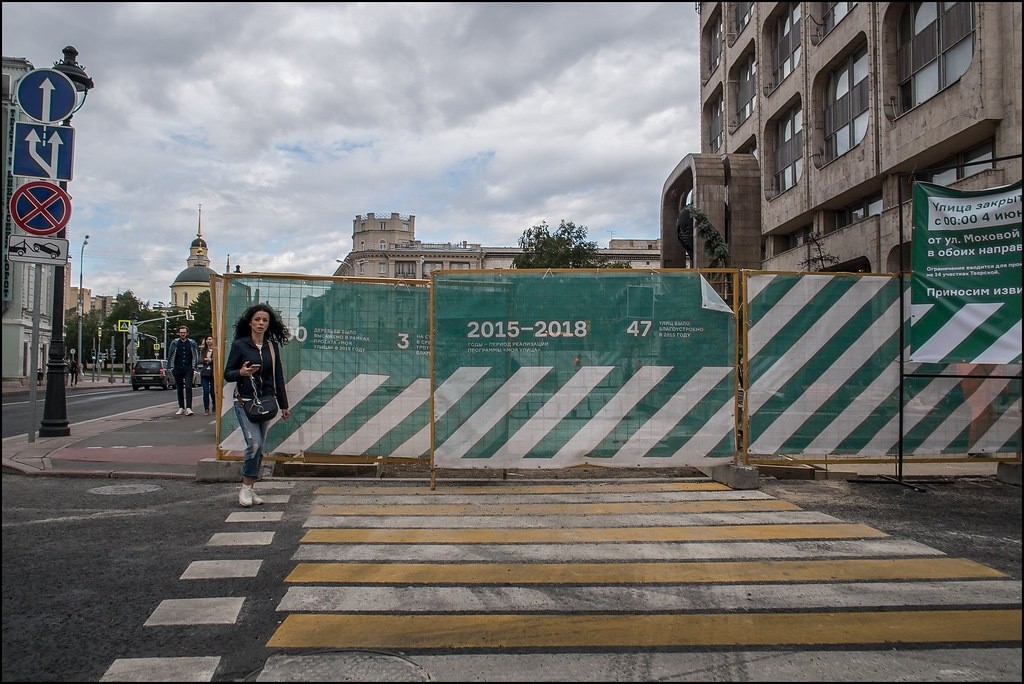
<box><xmin>258</xmin><ymin>402</ymin><xmax>261</xmax><ymax>404</ymax></box>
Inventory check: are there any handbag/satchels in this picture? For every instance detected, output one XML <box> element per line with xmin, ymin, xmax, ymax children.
<box><xmin>245</xmin><ymin>394</ymin><xmax>279</xmax><ymax>422</ymax></box>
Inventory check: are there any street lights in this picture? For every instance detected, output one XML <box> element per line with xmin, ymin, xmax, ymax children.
<box><xmin>129</xmin><ymin>312</ymin><xmax>140</xmax><ymax>383</ymax></box>
<box><xmin>158</xmin><ymin>299</ymin><xmax>173</xmax><ymax>360</ymax></box>
<box><xmin>77</xmin><ymin>235</ymin><xmax>91</xmax><ymax>380</ymax></box>
<box><xmin>38</xmin><ymin>46</ymin><xmax>95</xmax><ymax>439</ymax></box>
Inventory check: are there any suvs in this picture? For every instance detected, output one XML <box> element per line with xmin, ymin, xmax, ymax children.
<box><xmin>133</xmin><ymin>358</ymin><xmax>181</xmax><ymax>391</ymax></box>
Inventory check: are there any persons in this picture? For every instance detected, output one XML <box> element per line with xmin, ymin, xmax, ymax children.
<box><xmin>224</xmin><ymin>303</ymin><xmax>290</xmax><ymax>506</ymax></box>
<box><xmin>166</xmin><ymin>325</ymin><xmax>198</xmax><ymax>417</ymax></box>
<box><xmin>65</xmin><ymin>357</ymin><xmax>78</xmax><ymax>388</ymax></box>
<box><xmin>199</xmin><ymin>336</ymin><xmax>216</xmax><ymax>416</ymax></box>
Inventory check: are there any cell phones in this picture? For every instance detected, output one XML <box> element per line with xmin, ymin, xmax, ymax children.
<box><xmin>249</xmin><ymin>364</ymin><xmax>261</xmax><ymax>368</ymax></box>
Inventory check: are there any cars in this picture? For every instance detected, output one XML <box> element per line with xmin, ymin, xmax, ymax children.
<box><xmin>167</xmin><ymin>368</ymin><xmax>202</xmax><ymax>390</ymax></box>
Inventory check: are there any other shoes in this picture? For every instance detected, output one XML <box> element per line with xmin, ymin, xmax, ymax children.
<box><xmin>203</xmin><ymin>409</ymin><xmax>215</xmax><ymax>416</ymax></box>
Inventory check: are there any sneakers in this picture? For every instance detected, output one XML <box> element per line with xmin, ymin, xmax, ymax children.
<box><xmin>176</xmin><ymin>408</ymin><xmax>184</xmax><ymax>415</ymax></box>
<box><xmin>238</xmin><ymin>489</ymin><xmax>263</xmax><ymax>507</ymax></box>
<box><xmin>185</xmin><ymin>408</ymin><xmax>194</xmax><ymax>416</ymax></box>
